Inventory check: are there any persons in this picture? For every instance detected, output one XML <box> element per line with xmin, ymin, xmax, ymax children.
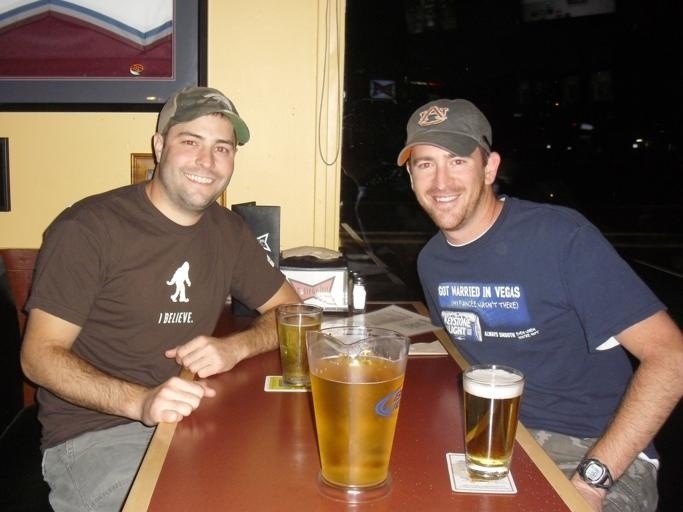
<box><xmin>20</xmin><ymin>86</ymin><xmax>302</xmax><ymax>511</ymax></box>
<box><xmin>398</xmin><ymin>96</ymin><xmax>683</xmax><ymax>511</ymax></box>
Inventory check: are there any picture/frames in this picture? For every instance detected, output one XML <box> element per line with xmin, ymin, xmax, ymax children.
<box><xmin>0</xmin><ymin>137</ymin><xmax>11</xmax><ymax>213</ymax></box>
<box><xmin>278</xmin><ymin>265</ymin><xmax>349</xmax><ymax>313</ymax></box>
<box><xmin>0</xmin><ymin>0</ymin><xmax>208</xmax><ymax>114</ymax></box>
<box><xmin>130</xmin><ymin>152</ymin><xmax>227</xmax><ymax>208</ymax></box>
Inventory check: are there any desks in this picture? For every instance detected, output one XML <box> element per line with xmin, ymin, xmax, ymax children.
<box><xmin>115</xmin><ymin>298</ymin><xmax>600</xmax><ymax>512</ymax></box>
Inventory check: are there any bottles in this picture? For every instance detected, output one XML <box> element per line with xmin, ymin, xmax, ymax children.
<box><xmin>348</xmin><ymin>265</ymin><xmax>369</xmax><ymax>315</ymax></box>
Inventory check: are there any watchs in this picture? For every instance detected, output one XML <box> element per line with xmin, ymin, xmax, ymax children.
<box><xmin>576</xmin><ymin>457</ymin><xmax>615</xmax><ymax>492</ymax></box>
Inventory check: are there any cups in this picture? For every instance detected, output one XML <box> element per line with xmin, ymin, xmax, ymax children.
<box><xmin>305</xmin><ymin>326</ymin><xmax>412</xmax><ymax>504</ymax></box>
<box><xmin>273</xmin><ymin>302</ymin><xmax>326</xmax><ymax>389</ymax></box>
<box><xmin>460</xmin><ymin>363</ymin><xmax>527</xmax><ymax>482</ymax></box>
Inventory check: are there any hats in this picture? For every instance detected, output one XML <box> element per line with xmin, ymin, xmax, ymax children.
<box><xmin>397</xmin><ymin>97</ymin><xmax>491</xmax><ymax>166</ymax></box>
<box><xmin>155</xmin><ymin>84</ymin><xmax>251</xmax><ymax>146</ymax></box>
